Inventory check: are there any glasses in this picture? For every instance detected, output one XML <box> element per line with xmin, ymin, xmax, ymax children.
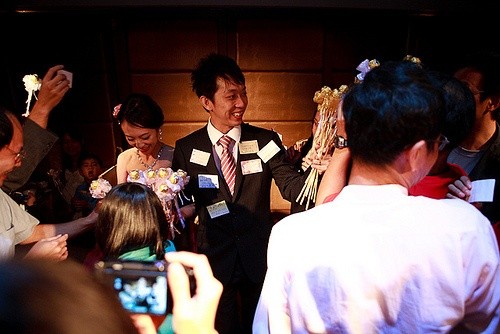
<box><xmin>5</xmin><ymin>146</ymin><xmax>26</xmax><ymax>162</ymax></box>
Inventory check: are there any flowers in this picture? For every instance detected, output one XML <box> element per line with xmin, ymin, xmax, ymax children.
<box><xmin>89</xmin><ymin>178</ymin><xmax>111</xmax><ymax>199</ymax></box>
<box><xmin>296</xmin><ymin>85</ymin><xmax>358</xmax><ymax>212</ymax></box>
<box><xmin>127</xmin><ymin>167</ymin><xmax>190</xmax><ymax>238</ymax></box>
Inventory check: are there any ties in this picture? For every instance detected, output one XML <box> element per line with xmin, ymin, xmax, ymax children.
<box><xmin>219</xmin><ymin>137</ymin><xmax>237</xmax><ymax>196</ymax></box>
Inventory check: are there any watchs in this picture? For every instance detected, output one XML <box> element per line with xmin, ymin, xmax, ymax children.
<box><xmin>333</xmin><ymin>135</ymin><xmax>349</xmax><ymax>148</ymax></box>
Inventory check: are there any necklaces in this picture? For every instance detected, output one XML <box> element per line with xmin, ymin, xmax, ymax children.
<box><xmin>137</xmin><ymin>145</ymin><xmax>163</xmax><ymax>172</ymax></box>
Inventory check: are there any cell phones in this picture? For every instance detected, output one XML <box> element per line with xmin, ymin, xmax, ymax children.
<box><xmin>57</xmin><ymin>70</ymin><xmax>72</xmax><ymax>88</ymax></box>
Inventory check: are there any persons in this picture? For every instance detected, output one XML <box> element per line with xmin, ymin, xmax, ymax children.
<box><xmin>0</xmin><ymin>252</ymin><xmax>223</xmax><ymax>334</ymax></box>
<box><xmin>0</xmin><ymin>65</ymin><xmax>103</xmax><ymax>262</ymax></box>
<box><xmin>113</xmin><ymin>94</ymin><xmax>195</xmax><ymax>239</ymax></box>
<box><xmin>252</xmin><ymin>61</ymin><xmax>499</xmax><ymax>334</ymax></box>
<box><xmin>86</xmin><ymin>181</ymin><xmax>175</xmax><ymax>334</ymax></box>
<box><xmin>171</xmin><ymin>52</ymin><xmax>331</xmax><ymax>334</ymax></box>
<box><xmin>286</xmin><ymin>63</ymin><xmax>500</xmax><ymax>247</ymax></box>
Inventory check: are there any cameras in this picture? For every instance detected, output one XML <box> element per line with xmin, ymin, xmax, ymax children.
<box><xmin>94</xmin><ymin>259</ymin><xmax>196</xmax><ymax>314</ymax></box>
